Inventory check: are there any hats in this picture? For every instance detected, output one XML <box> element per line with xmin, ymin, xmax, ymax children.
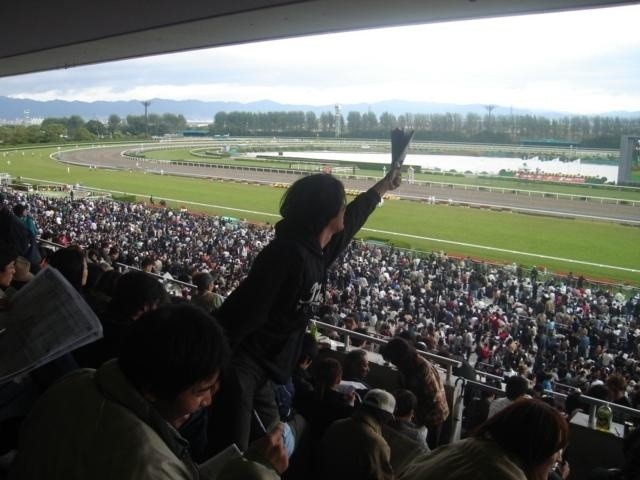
<box><xmin>361</xmin><ymin>388</ymin><xmax>397</xmax><ymax>422</ymax></box>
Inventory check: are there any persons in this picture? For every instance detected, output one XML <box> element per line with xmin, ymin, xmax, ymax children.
<box><xmin>0</xmin><ymin>167</ymin><xmax>640</xmax><ymax>480</ymax></box>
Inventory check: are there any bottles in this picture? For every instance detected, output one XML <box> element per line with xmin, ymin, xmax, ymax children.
<box><xmin>309</xmin><ymin>320</ymin><xmax>317</xmax><ymax>339</ymax></box>
<box><xmin>596</xmin><ymin>402</ymin><xmax>613</xmax><ymax>434</ymax></box>
